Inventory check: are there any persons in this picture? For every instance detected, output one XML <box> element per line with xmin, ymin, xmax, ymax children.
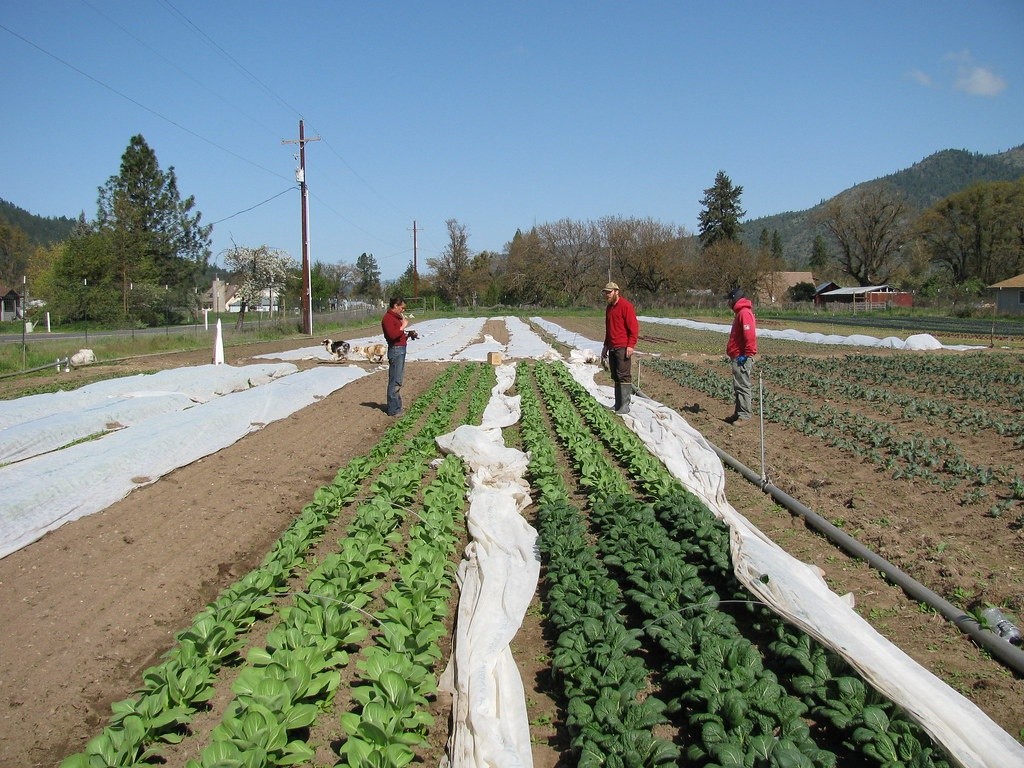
<box><xmin>601</xmin><ymin>283</ymin><xmax>638</xmax><ymax>415</ymax></box>
<box><xmin>722</xmin><ymin>290</ymin><xmax>756</xmax><ymax>426</ymax></box>
<box><xmin>382</xmin><ymin>296</ymin><xmax>418</xmax><ymax>416</ymax></box>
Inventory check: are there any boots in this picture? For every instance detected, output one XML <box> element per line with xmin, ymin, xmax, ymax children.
<box><xmin>609</xmin><ymin>382</ymin><xmax>622</xmax><ymax>411</ymax></box>
<box><xmin>615</xmin><ymin>383</ymin><xmax>632</xmax><ymax>414</ymax></box>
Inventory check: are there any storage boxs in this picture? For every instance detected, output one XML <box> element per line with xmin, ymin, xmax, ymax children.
<box><xmin>487</xmin><ymin>351</ymin><xmax>502</xmax><ymax>365</ymax></box>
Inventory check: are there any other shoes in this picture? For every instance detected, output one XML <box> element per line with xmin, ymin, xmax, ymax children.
<box><xmin>400</xmin><ymin>408</ymin><xmax>409</xmax><ymax>414</ymax></box>
<box><xmin>725</xmin><ymin>414</ymin><xmax>739</xmax><ymax>423</ymax></box>
<box><xmin>393</xmin><ymin>412</ymin><xmax>405</xmax><ymax>418</ymax></box>
<box><xmin>732</xmin><ymin>418</ymin><xmax>751</xmax><ymax>427</ymax></box>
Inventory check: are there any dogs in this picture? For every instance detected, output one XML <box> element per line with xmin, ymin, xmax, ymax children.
<box><xmin>321</xmin><ymin>338</ymin><xmax>388</xmax><ymax>364</ymax></box>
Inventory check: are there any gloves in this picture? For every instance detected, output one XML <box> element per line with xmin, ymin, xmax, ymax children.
<box><xmin>736</xmin><ymin>356</ymin><xmax>747</xmax><ymax>366</ymax></box>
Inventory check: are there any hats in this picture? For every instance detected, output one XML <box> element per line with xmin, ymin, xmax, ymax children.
<box><xmin>601</xmin><ymin>282</ymin><xmax>619</xmax><ymax>292</ymax></box>
<box><xmin>723</xmin><ymin>290</ymin><xmax>742</xmax><ymax>303</ymax></box>
